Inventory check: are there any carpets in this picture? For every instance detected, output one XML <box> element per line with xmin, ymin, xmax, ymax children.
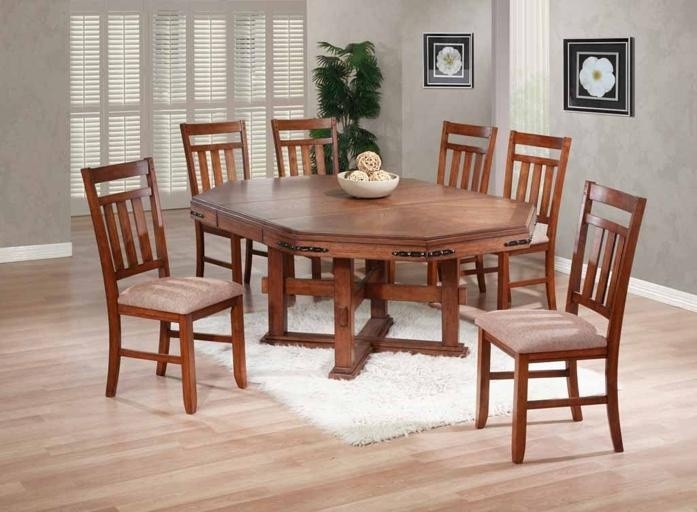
<box><xmin>170</xmin><ymin>298</ymin><xmax>621</xmax><ymax>446</ymax></box>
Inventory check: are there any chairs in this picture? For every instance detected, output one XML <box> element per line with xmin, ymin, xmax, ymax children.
<box><xmin>272</xmin><ymin>117</ymin><xmax>339</xmax><ymax>301</ymax></box>
<box><xmin>387</xmin><ymin>121</ymin><xmax>498</xmax><ymax>303</ymax></box>
<box><xmin>460</xmin><ymin>130</ymin><xmax>572</xmax><ymax>310</ymax></box>
<box><xmin>475</xmin><ymin>181</ymin><xmax>647</xmax><ymax>464</ymax></box>
<box><xmin>81</xmin><ymin>157</ymin><xmax>247</xmax><ymax>414</ymax></box>
<box><xmin>180</xmin><ymin>119</ymin><xmax>268</xmax><ymax>286</ymax></box>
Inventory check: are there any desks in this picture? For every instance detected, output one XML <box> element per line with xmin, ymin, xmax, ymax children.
<box><xmin>189</xmin><ymin>175</ymin><xmax>537</xmax><ymax>380</ymax></box>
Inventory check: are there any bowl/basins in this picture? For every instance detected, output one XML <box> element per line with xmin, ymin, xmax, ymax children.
<box><xmin>334</xmin><ymin>170</ymin><xmax>401</xmax><ymax>199</ymax></box>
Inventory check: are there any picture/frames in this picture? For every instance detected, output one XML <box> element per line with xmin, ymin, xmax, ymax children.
<box><xmin>422</xmin><ymin>32</ymin><xmax>474</xmax><ymax>89</ymax></box>
<box><xmin>562</xmin><ymin>37</ymin><xmax>633</xmax><ymax>117</ymax></box>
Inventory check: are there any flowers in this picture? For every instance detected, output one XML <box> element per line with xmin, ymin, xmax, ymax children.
<box><xmin>580</xmin><ymin>57</ymin><xmax>616</xmax><ymax>98</ymax></box>
<box><xmin>435</xmin><ymin>47</ymin><xmax>462</xmax><ymax>75</ymax></box>
